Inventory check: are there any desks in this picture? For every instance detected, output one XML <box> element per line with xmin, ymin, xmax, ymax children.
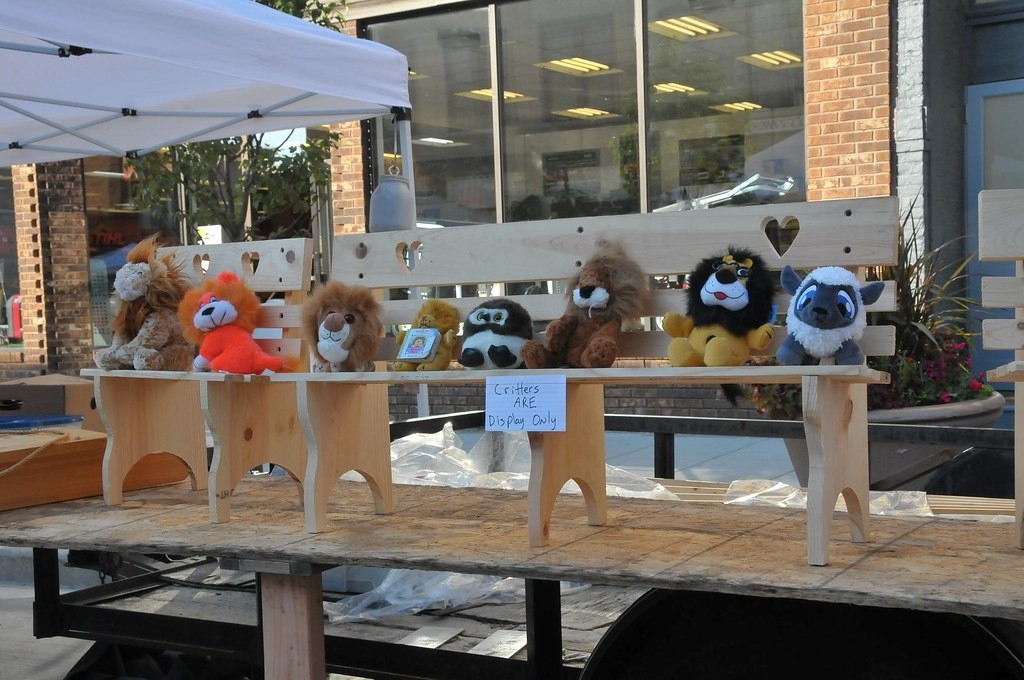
<box><xmin>0</xmin><ymin>473</ymin><xmax>1024</xmax><ymax>680</ymax></box>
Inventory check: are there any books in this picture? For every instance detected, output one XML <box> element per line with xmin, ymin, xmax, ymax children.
<box><xmin>397</xmin><ymin>328</ymin><xmax>442</xmax><ymax>363</ymax></box>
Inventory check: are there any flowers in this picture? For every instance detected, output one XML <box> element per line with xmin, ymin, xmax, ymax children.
<box><xmin>745</xmin><ymin>187</ymin><xmax>993</xmax><ymax>420</ymax></box>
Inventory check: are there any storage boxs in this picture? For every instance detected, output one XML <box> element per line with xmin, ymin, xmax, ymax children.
<box><xmin>0</xmin><ymin>373</ymin><xmax>108</xmax><ymax>433</ymax></box>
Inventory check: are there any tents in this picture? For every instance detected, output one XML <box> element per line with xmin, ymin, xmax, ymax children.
<box><xmin>0</xmin><ymin>0</ymin><xmax>429</xmax><ymax>418</ymax></box>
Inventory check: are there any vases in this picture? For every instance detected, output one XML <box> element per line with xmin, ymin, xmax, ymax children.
<box><xmin>785</xmin><ymin>392</ymin><xmax>1006</xmax><ymax>492</ymax></box>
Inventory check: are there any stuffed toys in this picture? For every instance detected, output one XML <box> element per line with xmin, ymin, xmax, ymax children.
<box><xmin>662</xmin><ymin>244</ymin><xmax>775</xmax><ymax>367</ymax></box>
<box><xmin>300</xmin><ymin>280</ymin><xmax>386</xmax><ymax>374</ymax></box>
<box><xmin>520</xmin><ymin>237</ymin><xmax>651</xmax><ymax>368</ymax></box>
<box><xmin>177</xmin><ymin>272</ymin><xmax>304</xmax><ymax>375</ymax></box>
<box><xmin>458</xmin><ymin>299</ymin><xmax>532</xmax><ymax>369</ymax></box>
<box><xmin>392</xmin><ymin>299</ymin><xmax>461</xmax><ymax>371</ymax></box>
<box><xmin>775</xmin><ymin>264</ymin><xmax>885</xmax><ymax>366</ymax></box>
<box><xmin>95</xmin><ymin>231</ymin><xmax>195</xmax><ymax>372</ymax></box>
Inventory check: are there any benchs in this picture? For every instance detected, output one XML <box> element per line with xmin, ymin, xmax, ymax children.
<box><xmin>80</xmin><ymin>237</ymin><xmax>315</xmax><ymax>524</ymax></box>
<box><xmin>977</xmin><ymin>189</ymin><xmax>1024</xmax><ymax>549</ymax></box>
<box><xmin>269</xmin><ymin>195</ymin><xmax>900</xmax><ymax>568</ymax></box>
